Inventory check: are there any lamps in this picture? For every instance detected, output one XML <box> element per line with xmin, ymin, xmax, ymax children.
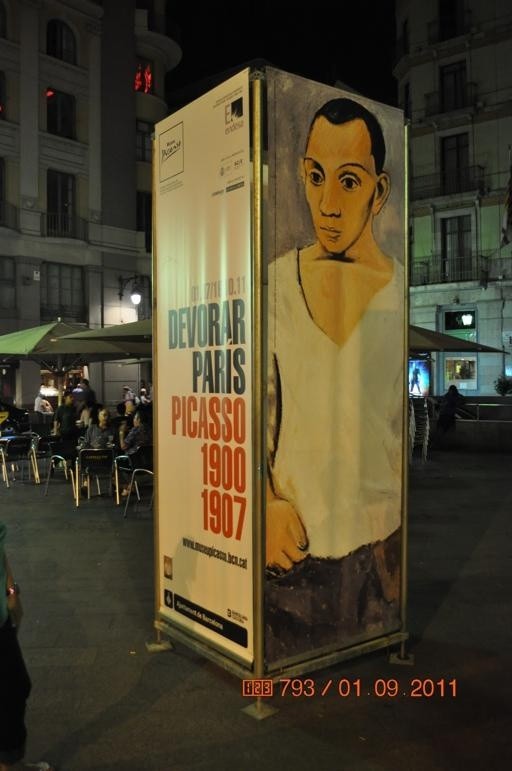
<box><xmin>118</xmin><ymin>274</ymin><xmax>141</xmax><ymax>305</ymax></box>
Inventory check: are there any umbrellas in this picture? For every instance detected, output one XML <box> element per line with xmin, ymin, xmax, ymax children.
<box><xmin>408</xmin><ymin>325</ymin><xmax>510</xmax><ymax>354</ymax></box>
<box><xmin>55</xmin><ymin>319</ymin><xmax>152</xmax><ymax>343</ymax></box>
<box><xmin>0</xmin><ymin>317</ymin><xmax>129</xmax><ymax>408</ymax></box>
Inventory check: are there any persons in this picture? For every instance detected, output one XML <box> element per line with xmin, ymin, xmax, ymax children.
<box><xmin>34</xmin><ymin>376</ymin><xmax>153</xmax><ymax>498</ymax></box>
<box><xmin>0</xmin><ymin>522</ymin><xmax>55</xmax><ymax>771</ymax></box>
<box><xmin>432</xmin><ymin>386</ymin><xmax>464</xmax><ymax>447</ymax></box>
<box><xmin>266</xmin><ymin>98</ymin><xmax>406</xmax><ymax>661</ymax></box>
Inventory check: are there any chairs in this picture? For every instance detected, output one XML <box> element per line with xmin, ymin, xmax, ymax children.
<box><xmin>0</xmin><ymin>408</ymin><xmax>153</xmax><ymax>518</ymax></box>
<box><xmin>408</xmin><ymin>395</ymin><xmax>430</xmax><ymax>467</ymax></box>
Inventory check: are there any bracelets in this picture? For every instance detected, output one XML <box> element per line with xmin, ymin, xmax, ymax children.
<box><xmin>7</xmin><ymin>583</ymin><xmax>20</xmax><ymax>596</ymax></box>
